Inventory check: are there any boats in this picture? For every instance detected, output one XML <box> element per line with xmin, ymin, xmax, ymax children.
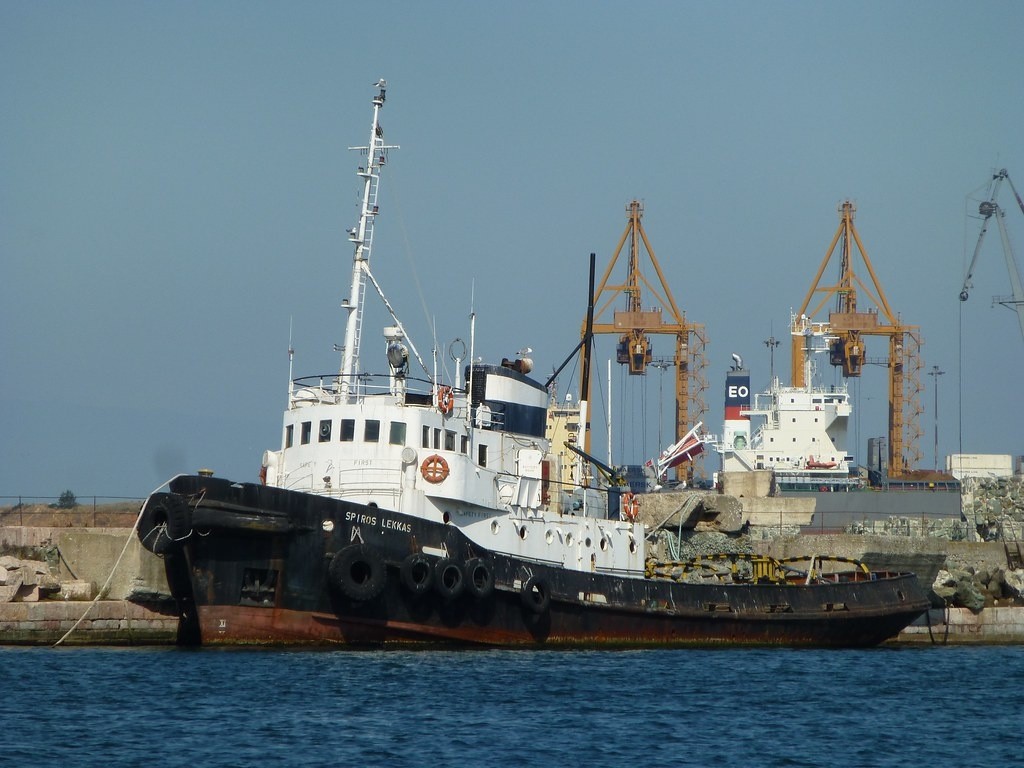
<box><xmin>136</xmin><ymin>76</ymin><xmax>990</xmax><ymax>647</ymax></box>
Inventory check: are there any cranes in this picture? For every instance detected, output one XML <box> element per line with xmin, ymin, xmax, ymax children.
<box><xmin>788</xmin><ymin>200</ymin><xmax>926</xmax><ymax>484</ymax></box>
<box><xmin>577</xmin><ymin>198</ymin><xmax>711</xmax><ymax>487</ymax></box>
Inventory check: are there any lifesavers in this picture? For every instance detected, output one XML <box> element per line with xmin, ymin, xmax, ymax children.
<box><xmin>326</xmin><ymin>543</ymin><xmax>390</xmax><ymax>604</ymax></box>
<box><xmin>520</xmin><ymin>577</ymin><xmax>551</xmax><ymax>614</ymax></box>
<box><xmin>422</xmin><ymin>455</ymin><xmax>448</xmax><ymax>483</ymax></box>
<box><xmin>462</xmin><ymin>556</ymin><xmax>496</xmax><ymax>598</ymax></box>
<box><xmin>432</xmin><ymin>557</ymin><xmax>464</xmax><ymax>596</ymax></box>
<box><xmin>623</xmin><ymin>493</ymin><xmax>639</xmax><ymax>519</ymax></box>
<box><xmin>400</xmin><ymin>552</ymin><xmax>433</xmax><ymax>592</ymax></box>
<box><xmin>439</xmin><ymin>385</ymin><xmax>454</xmax><ymax>414</ymax></box>
<box><xmin>135</xmin><ymin>492</ymin><xmax>190</xmax><ymax>555</ymax></box>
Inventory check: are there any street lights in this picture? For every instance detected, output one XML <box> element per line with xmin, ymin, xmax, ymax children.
<box><xmin>762</xmin><ymin>336</ymin><xmax>782</xmax><ymax>411</ymax></box>
<box><xmin>927</xmin><ymin>366</ymin><xmax>946</xmax><ymax>473</ymax></box>
